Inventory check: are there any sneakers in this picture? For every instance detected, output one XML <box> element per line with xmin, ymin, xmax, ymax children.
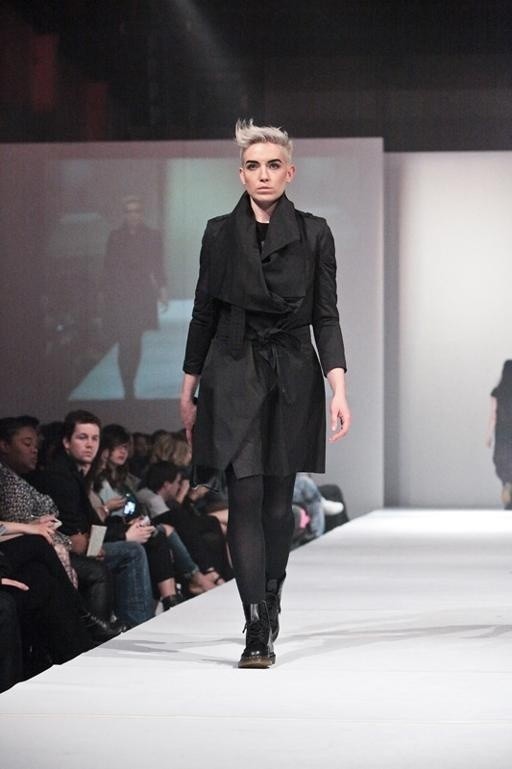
<box><xmin>323</xmin><ymin>500</ymin><xmax>344</xmax><ymax>516</ymax></box>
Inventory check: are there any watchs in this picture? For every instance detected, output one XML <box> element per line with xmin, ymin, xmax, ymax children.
<box><xmin>102</xmin><ymin>505</ymin><xmax>109</xmax><ymax>515</ymax></box>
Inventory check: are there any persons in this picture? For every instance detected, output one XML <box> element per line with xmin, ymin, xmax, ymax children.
<box><xmin>1</xmin><ymin>412</ymin><xmax>228</xmax><ymax>688</ymax></box>
<box><xmin>179</xmin><ymin>117</ymin><xmax>351</xmax><ymax>665</ymax></box>
<box><xmin>293</xmin><ymin>469</ymin><xmax>348</xmax><ymax>547</ymax></box>
<box><xmin>104</xmin><ymin>198</ymin><xmax>171</xmax><ymax>398</ymax></box>
<box><xmin>487</xmin><ymin>357</ymin><xmax>512</xmax><ymax>510</ymax></box>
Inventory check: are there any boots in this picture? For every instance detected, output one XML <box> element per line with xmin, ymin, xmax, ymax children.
<box><xmin>238</xmin><ymin>598</ymin><xmax>275</xmax><ymax>668</ymax></box>
<box><xmin>80</xmin><ymin>612</ymin><xmax>127</xmax><ymax>648</ymax></box>
<box><xmin>265</xmin><ymin>572</ymin><xmax>286</xmax><ymax>641</ymax></box>
